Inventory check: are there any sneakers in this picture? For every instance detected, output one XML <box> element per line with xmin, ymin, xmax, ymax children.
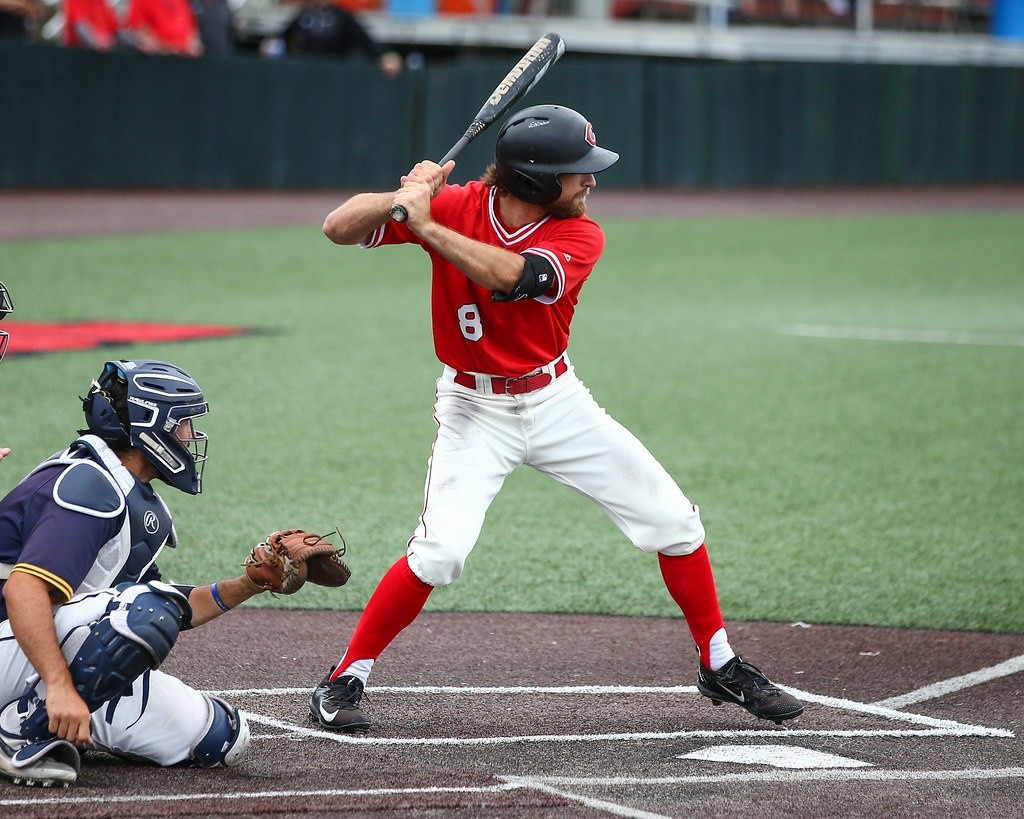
<box><xmin>697</xmin><ymin>654</ymin><xmax>804</xmax><ymax>724</ymax></box>
<box><xmin>0</xmin><ymin>745</ymin><xmax>76</xmax><ymax>788</ymax></box>
<box><xmin>309</xmin><ymin>665</ymin><xmax>372</xmax><ymax>730</ymax></box>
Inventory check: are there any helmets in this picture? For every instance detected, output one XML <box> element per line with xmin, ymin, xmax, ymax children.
<box><xmin>77</xmin><ymin>359</ymin><xmax>210</xmax><ymax>495</ymax></box>
<box><xmin>495</xmin><ymin>104</ymin><xmax>619</xmax><ymax>204</ymax></box>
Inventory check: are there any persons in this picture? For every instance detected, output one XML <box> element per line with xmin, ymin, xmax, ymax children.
<box><xmin>0</xmin><ymin>2</ymin><xmax>997</xmax><ymax>63</ymax></box>
<box><xmin>0</xmin><ymin>282</ymin><xmax>351</xmax><ymax>787</ymax></box>
<box><xmin>308</xmin><ymin>103</ymin><xmax>805</xmax><ymax>733</ymax></box>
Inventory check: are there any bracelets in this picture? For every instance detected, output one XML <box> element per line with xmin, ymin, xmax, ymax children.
<box><xmin>211</xmin><ymin>582</ymin><xmax>230</xmax><ymax>612</ymax></box>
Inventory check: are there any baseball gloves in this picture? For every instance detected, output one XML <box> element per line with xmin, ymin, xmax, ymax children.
<box><xmin>244</xmin><ymin>529</ymin><xmax>354</xmax><ymax>596</ymax></box>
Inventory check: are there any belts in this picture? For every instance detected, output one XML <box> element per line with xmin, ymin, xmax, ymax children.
<box><xmin>454</xmin><ymin>357</ymin><xmax>568</xmax><ymax>395</ymax></box>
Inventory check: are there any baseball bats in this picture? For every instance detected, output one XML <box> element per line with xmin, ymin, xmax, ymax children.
<box><xmin>389</xmin><ymin>30</ymin><xmax>568</xmax><ymax>223</ymax></box>
